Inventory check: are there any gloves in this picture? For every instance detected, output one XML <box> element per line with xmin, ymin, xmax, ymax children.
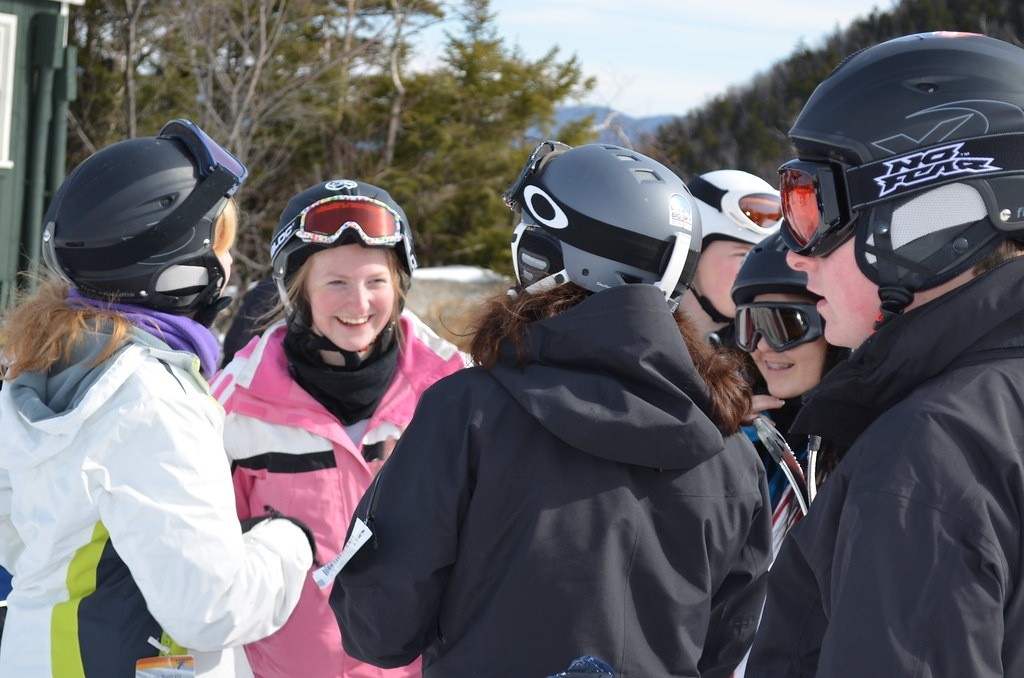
<box><xmin>241</xmin><ymin>504</ymin><xmax>315</xmax><ymax>554</ymax></box>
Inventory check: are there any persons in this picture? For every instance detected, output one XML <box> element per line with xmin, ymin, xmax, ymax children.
<box><xmin>675</xmin><ymin>32</ymin><xmax>1024</xmax><ymax>678</ymax></box>
<box><xmin>329</xmin><ymin>144</ymin><xmax>773</xmax><ymax>678</ymax></box>
<box><xmin>0</xmin><ymin>119</ymin><xmax>316</xmax><ymax>678</ymax></box>
<box><xmin>207</xmin><ymin>179</ymin><xmax>483</xmax><ymax>678</ymax></box>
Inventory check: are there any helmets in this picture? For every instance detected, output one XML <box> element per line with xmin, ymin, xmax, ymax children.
<box><xmin>731</xmin><ymin>231</ymin><xmax>823</xmax><ymax>304</ymax></box>
<box><xmin>512</xmin><ymin>143</ymin><xmax>702</xmax><ymax>313</ymax></box>
<box><xmin>789</xmin><ymin>28</ymin><xmax>1024</xmax><ymax>292</ymax></box>
<box><xmin>40</xmin><ymin>136</ymin><xmax>225</xmax><ymax>314</ymax></box>
<box><xmin>270</xmin><ymin>180</ymin><xmax>417</xmax><ymax>327</ymax></box>
<box><xmin>687</xmin><ymin>170</ymin><xmax>784</xmax><ymax>257</ymax></box>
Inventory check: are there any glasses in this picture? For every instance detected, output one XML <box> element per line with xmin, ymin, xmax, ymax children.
<box><xmin>778</xmin><ymin>158</ymin><xmax>852</xmax><ymax>257</ymax></box>
<box><xmin>734</xmin><ymin>302</ymin><xmax>823</xmax><ymax>352</ymax></box>
<box><xmin>296</xmin><ymin>195</ymin><xmax>402</xmax><ymax>247</ymax></box>
<box><xmin>721</xmin><ymin>186</ymin><xmax>784</xmax><ymax>233</ymax></box>
<box><xmin>179</xmin><ymin>117</ymin><xmax>249</xmax><ymax>183</ymax></box>
